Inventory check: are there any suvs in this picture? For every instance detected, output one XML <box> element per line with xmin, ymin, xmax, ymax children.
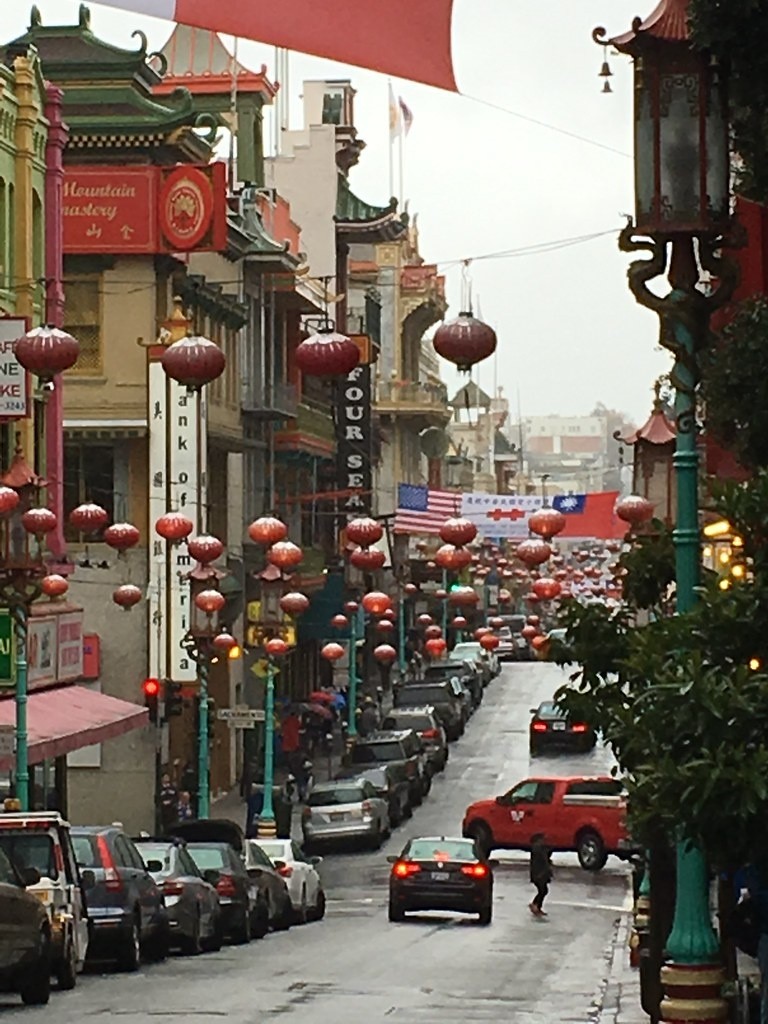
<box><xmin>128</xmin><ymin>834</ymin><xmax>224</xmax><ymax>955</ymax></box>
<box><xmin>67</xmin><ymin>821</ymin><xmax>170</xmax><ymax>973</ymax></box>
<box><xmin>164</xmin><ymin>815</ymin><xmax>326</xmax><ymax>944</ymax></box>
<box><xmin>337</xmin><ymin>612</ymin><xmax>584</xmax><ymax>829</ymax></box>
<box><xmin>299</xmin><ymin>776</ymin><xmax>395</xmax><ymax>856</ymax></box>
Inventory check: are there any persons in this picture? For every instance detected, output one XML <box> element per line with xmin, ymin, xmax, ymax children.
<box><xmin>240</xmin><ymin>645</ymin><xmax>424</xmax><ymax>802</ymax></box>
<box><xmin>528</xmin><ymin>832</ymin><xmax>554</xmax><ymax>918</ymax></box>
<box><xmin>282</xmin><ymin>774</ymin><xmax>300</xmax><ymax>830</ymax></box>
<box><xmin>180</xmin><ymin>759</ymin><xmax>211</xmax><ymax>803</ymax></box>
<box><xmin>156</xmin><ymin>772</ymin><xmax>178</xmax><ymax>821</ymax></box>
<box><xmin>244</xmin><ymin>783</ymin><xmax>264</xmax><ymax>840</ymax></box>
<box><xmin>175</xmin><ymin>790</ymin><xmax>196</xmax><ymax>822</ymax></box>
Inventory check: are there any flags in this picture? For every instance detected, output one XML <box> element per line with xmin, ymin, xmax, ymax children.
<box><xmin>394</xmin><ymin>483</ymin><xmax>461</xmax><ymax>533</ymax></box>
<box><xmin>549</xmin><ymin>491</ymin><xmax>632</xmax><ymax>539</ymax></box>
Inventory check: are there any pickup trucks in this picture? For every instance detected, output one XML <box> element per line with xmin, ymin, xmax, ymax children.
<box><xmin>1</xmin><ymin>810</ymin><xmax>97</xmax><ymax>990</ymax></box>
<box><xmin>461</xmin><ymin>773</ymin><xmax>639</xmax><ymax>871</ymax></box>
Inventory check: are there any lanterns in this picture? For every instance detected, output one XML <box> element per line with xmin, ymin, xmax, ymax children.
<box><xmin>15</xmin><ymin>320</ymin><xmax>80</xmax><ymax>391</ymax></box>
<box><xmin>44</xmin><ymin>575</ymin><xmax>561</xmax><ymax>661</ymax></box>
<box><xmin>0</xmin><ymin>484</ymin><xmax>652</xmax><ymax>574</ymax></box>
<box><xmin>561</xmin><ymin>570</ymin><xmax>590</xmax><ymax>604</ymax></box>
<box><xmin>293</xmin><ymin>326</ymin><xmax>361</xmax><ymax>387</ymax></box>
<box><xmin>431</xmin><ymin>311</ymin><xmax>498</xmax><ymax>374</ymax></box>
<box><xmin>162</xmin><ymin>331</ymin><xmax>225</xmax><ymax>392</ymax></box>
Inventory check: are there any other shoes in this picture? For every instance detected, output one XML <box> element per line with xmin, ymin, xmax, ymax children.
<box><xmin>531</xmin><ymin>902</ymin><xmax>543</xmax><ymax>918</ymax></box>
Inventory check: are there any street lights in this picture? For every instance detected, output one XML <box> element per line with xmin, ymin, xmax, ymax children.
<box><xmin>175</xmin><ymin>560</ymin><xmax>229</xmax><ymax>820</ymax></box>
<box><xmin>606</xmin><ymin>382</ymin><xmax>680</xmax><ymax>966</ymax></box>
<box><xmin>338</xmin><ymin>538</ymin><xmax>370</xmax><ymax>756</ymax></box>
<box><xmin>0</xmin><ymin>430</ymin><xmax>48</xmax><ymax>812</ymax></box>
<box><xmin>584</xmin><ymin>0</ymin><xmax>768</xmax><ymax>1023</ymax></box>
<box><xmin>389</xmin><ymin>528</ymin><xmax>417</xmax><ymax>681</ymax></box>
<box><xmin>248</xmin><ymin>550</ymin><xmax>297</xmax><ymax>840</ymax></box>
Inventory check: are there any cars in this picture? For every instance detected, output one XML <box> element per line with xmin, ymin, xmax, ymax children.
<box><xmin>385</xmin><ymin>835</ymin><xmax>501</xmax><ymax>927</ymax></box>
<box><xmin>527</xmin><ymin>699</ymin><xmax>596</xmax><ymax>756</ymax></box>
<box><xmin>0</xmin><ymin>845</ymin><xmax>53</xmax><ymax>1006</ymax></box>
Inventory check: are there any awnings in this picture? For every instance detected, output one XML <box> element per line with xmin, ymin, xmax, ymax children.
<box><xmin>0</xmin><ymin>685</ymin><xmax>150</xmax><ymax>774</ymax></box>
